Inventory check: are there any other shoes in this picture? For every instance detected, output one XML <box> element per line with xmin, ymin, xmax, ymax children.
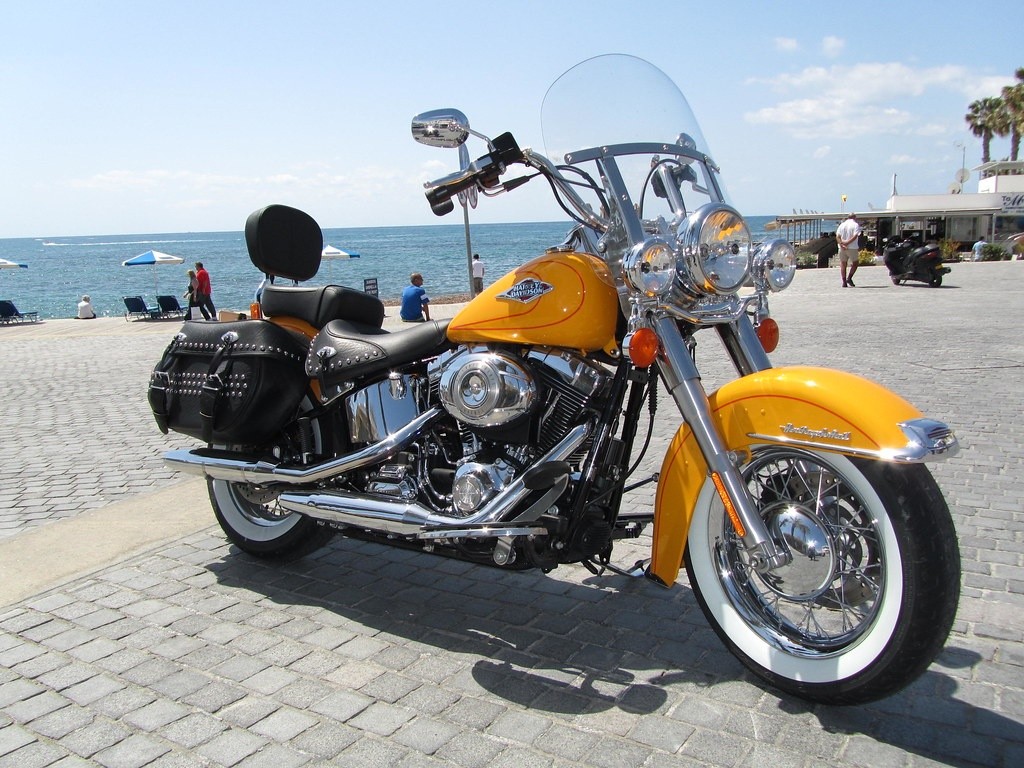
<box><xmin>845</xmin><ymin>279</ymin><xmax>855</xmax><ymax>286</ymax></box>
<box><xmin>842</xmin><ymin>283</ymin><xmax>847</xmax><ymax>287</ymax></box>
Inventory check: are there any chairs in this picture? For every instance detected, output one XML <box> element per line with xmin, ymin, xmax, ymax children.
<box><xmin>120</xmin><ymin>295</ymin><xmax>151</xmax><ymax>324</ymax></box>
<box><xmin>155</xmin><ymin>294</ymin><xmax>188</xmax><ymax>321</ymax></box>
<box><xmin>244</xmin><ymin>203</ymin><xmax>386</xmax><ymax>330</ymax></box>
<box><xmin>0</xmin><ymin>297</ymin><xmax>38</xmax><ymax>325</ymax></box>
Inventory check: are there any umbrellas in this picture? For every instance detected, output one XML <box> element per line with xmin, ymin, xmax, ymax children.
<box><xmin>122</xmin><ymin>251</ymin><xmax>185</xmax><ymax>310</ymax></box>
<box><xmin>322</xmin><ymin>244</ymin><xmax>360</xmax><ymax>285</ymax></box>
<box><xmin>0</xmin><ymin>259</ymin><xmax>28</xmax><ymax>269</ymax></box>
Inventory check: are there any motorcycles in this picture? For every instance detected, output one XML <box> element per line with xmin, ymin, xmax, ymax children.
<box><xmin>882</xmin><ymin>232</ymin><xmax>952</xmax><ymax>289</ymax></box>
<box><xmin>148</xmin><ymin>53</ymin><xmax>962</xmax><ymax>709</ymax></box>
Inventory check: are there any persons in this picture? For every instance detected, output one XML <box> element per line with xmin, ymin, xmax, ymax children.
<box><xmin>858</xmin><ymin>231</ymin><xmax>864</xmax><ymax>247</ymax></box>
<box><xmin>970</xmin><ymin>236</ymin><xmax>988</xmax><ymax>262</ymax></box>
<box><xmin>473</xmin><ymin>254</ymin><xmax>485</xmax><ymax>296</ymax></box>
<box><xmin>182</xmin><ymin>270</ymin><xmax>213</xmax><ymax>323</ymax></box>
<box><xmin>195</xmin><ymin>262</ymin><xmax>217</xmax><ymax>320</ymax></box>
<box><xmin>820</xmin><ymin>232</ymin><xmax>836</xmax><ymax>238</ymax></box>
<box><xmin>78</xmin><ymin>295</ymin><xmax>96</xmax><ymax>319</ymax></box>
<box><xmin>399</xmin><ymin>273</ymin><xmax>433</xmax><ymax>322</ymax></box>
<box><xmin>836</xmin><ymin>213</ymin><xmax>860</xmax><ymax>287</ymax></box>
